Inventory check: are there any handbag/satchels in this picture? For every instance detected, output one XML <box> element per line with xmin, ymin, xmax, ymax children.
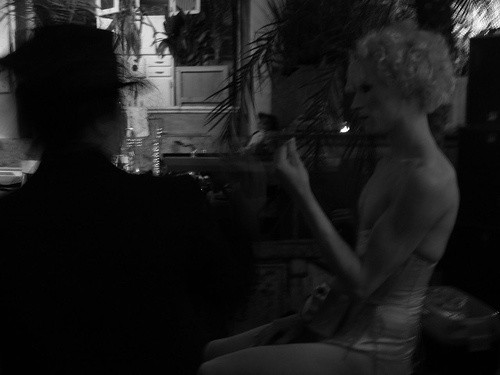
<box><xmin>292</xmin><ymin>269</ymin><xmax>356</xmax><ymax>338</ymax></box>
<box><xmin>421</xmin><ymin>285</ymin><xmax>500</xmax><ymax>352</ymax></box>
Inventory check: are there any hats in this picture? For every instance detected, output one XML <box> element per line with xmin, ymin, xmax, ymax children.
<box><xmin>0</xmin><ymin>21</ymin><xmax>124</xmax><ymax>136</ymax></box>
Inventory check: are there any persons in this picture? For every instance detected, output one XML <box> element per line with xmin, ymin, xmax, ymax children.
<box><xmin>0</xmin><ymin>23</ymin><xmax>252</xmax><ymax>375</ymax></box>
<box><xmin>200</xmin><ymin>24</ymin><xmax>462</xmax><ymax>374</ymax></box>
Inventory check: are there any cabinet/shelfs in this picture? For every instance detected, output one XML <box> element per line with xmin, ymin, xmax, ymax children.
<box><xmin>93</xmin><ymin>14</ymin><xmax>242</xmax><ymax>179</ymax></box>
